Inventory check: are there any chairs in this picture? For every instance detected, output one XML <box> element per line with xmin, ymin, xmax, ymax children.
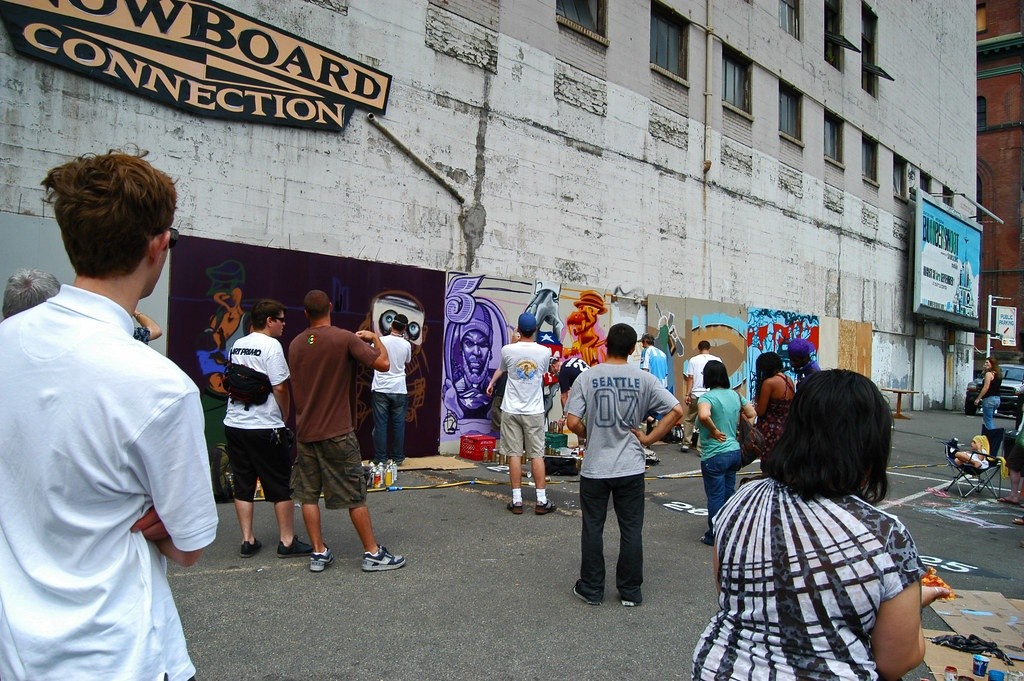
<box><xmin>940</xmin><ymin>425</ymin><xmax>1005</xmax><ymax>499</ymax></box>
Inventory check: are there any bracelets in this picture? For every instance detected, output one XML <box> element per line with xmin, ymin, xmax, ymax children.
<box><xmin>709</xmin><ymin>426</ymin><xmax>717</xmax><ymax>432</ymax></box>
<box><xmin>684</xmin><ymin>394</ymin><xmax>690</xmax><ymax>397</ymax></box>
<box><xmin>134</xmin><ymin>312</ymin><xmax>144</xmax><ymax>318</ymax></box>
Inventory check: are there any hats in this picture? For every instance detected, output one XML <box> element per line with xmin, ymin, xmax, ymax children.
<box><xmin>550</xmin><ymin>357</ymin><xmax>554</xmax><ymax>365</ymax></box>
<box><xmin>518</xmin><ymin>313</ymin><xmax>536</xmax><ymax>331</ymax></box>
<box><xmin>636</xmin><ymin>333</ymin><xmax>654</xmax><ymax>342</ymax></box>
<box><xmin>788</xmin><ymin>339</ymin><xmax>814</xmax><ymax>357</ymax></box>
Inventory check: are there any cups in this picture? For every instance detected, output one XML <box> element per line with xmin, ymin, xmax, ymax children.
<box><xmin>944</xmin><ymin>665</ymin><xmax>957</xmax><ymax>681</ymax></box>
<box><xmin>988</xmin><ymin>670</ymin><xmax>1005</xmax><ymax>681</ymax></box>
<box><xmin>973</xmin><ymin>654</ymin><xmax>990</xmax><ymax>677</ymax></box>
<box><xmin>1007</xmin><ymin>669</ymin><xmax>1023</xmax><ymax>681</ymax></box>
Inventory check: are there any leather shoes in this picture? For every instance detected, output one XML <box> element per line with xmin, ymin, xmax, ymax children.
<box><xmin>277</xmin><ymin>534</ymin><xmax>314</xmax><ymax>557</ymax></box>
<box><xmin>241</xmin><ymin>538</ymin><xmax>261</xmax><ymax>557</ymax></box>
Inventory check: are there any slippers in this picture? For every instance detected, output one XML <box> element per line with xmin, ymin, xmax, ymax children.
<box><xmin>1020</xmin><ymin>503</ymin><xmax>1024</xmax><ymax>508</ymax></box>
<box><xmin>924</xmin><ymin>487</ymin><xmax>942</xmax><ymax>493</ymax></box>
<box><xmin>997</xmin><ymin>497</ymin><xmax>1019</xmax><ymax>505</ymax></box>
<box><xmin>935</xmin><ymin>492</ymin><xmax>952</xmax><ymax>498</ymax></box>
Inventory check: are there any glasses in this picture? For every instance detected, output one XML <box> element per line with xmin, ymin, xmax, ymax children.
<box><xmin>154</xmin><ymin>228</ymin><xmax>179</xmax><ymax>249</ymax></box>
<box><xmin>270</xmin><ymin>316</ymin><xmax>285</xmax><ymax>323</ymax></box>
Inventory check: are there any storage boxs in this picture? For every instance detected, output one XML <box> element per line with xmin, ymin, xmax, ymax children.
<box><xmin>459</xmin><ymin>434</ymin><xmax>496</xmax><ymax>461</ymax></box>
<box><xmin>545</xmin><ymin>432</ymin><xmax>567</xmax><ymax>449</ymax></box>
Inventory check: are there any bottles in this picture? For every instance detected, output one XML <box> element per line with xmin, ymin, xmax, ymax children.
<box><xmin>378</xmin><ymin>461</ymin><xmax>398</xmax><ymax>486</ymax></box>
<box><xmin>550</xmin><ymin>421</ymin><xmax>563</xmax><ymax>434</ymax></box>
<box><xmin>546</xmin><ymin>445</ymin><xmax>559</xmax><ymax>455</ymax></box>
<box><xmin>493</xmin><ymin>449</ymin><xmax>510</xmax><ymax>464</ymax></box>
<box><xmin>369</xmin><ymin>461</ymin><xmax>380</xmax><ymax>488</ymax></box>
<box><xmin>576</xmin><ymin>448</ymin><xmax>585</xmax><ymax>470</ymax></box>
<box><xmin>483</xmin><ymin>449</ymin><xmax>488</xmax><ymax>463</ymax></box>
<box><xmin>522</xmin><ymin>450</ymin><xmax>526</xmax><ymax>465</ymax></box>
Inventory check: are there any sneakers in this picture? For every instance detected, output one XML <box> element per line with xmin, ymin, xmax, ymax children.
<box><xmin>362</xmin><ymin>544</ymin><xmax>405</xmax><ymax>570</ymax></box>
<box><xmin>507</xmin><ymin>500</ymin><xmax>523</xmax><ymax>513</ymax></box>
<box><xmin>309</xmin><ymin>542</ymin><xmax>334</xmax><ymax>571</ymax></box>
<box><xmin>535</xmin><ymin>499</ymin><xmax>557</xmax><ymax>513</ymax></box>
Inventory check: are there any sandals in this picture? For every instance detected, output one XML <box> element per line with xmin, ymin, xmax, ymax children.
<box><xmin>1012</xmin><ymin>518</ymin><xmax>1024</xmax><ymax>525</ymax></box>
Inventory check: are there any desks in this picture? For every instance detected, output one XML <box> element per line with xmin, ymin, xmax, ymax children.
<box><xmin>881</xmin><ymin>389</ymin><xmax>919</xmax><ymax>419</ymax></box>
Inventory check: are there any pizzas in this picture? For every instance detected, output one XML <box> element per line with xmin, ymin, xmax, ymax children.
<box><xmin>919</xmin><ymin>568</ymin><xmax>956</xmax><ymax>599</ymax></box>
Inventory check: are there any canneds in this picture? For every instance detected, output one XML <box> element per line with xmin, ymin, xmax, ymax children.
<box><xmin>944</xmin><ymin>666</ymin><xmax>958</xmax><ymax>681</ymax></box>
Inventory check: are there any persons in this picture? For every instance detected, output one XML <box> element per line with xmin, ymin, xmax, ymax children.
<box><xmin>697</xmin><ymin>360</ymin><xmax>757</xmax><ymax>546</ymax></box>
<box><xmin>0</xmin><ymin>149</ymin><xmax>219</xmax><ymax>680</ymax></box>
<box><xmin>369</xmin><ymin>314</ymin><xmax>412</xmax><ymax>466</ymax></box>
<box><xmin>688</xmin><ymin>368</ymin><xmax>950</xmax><ymax>681</ymax></box>
<box><xmin>995</xmin><ymin>373</ymin><xmax>1024</xmax><ymax>524</ymax></box>
<box><xmin>221</xmin><ymin>299</ymin><xmax>313</xmax><ymax>558</ymax></box>
<box><xmin>486</xmin><ymin>312</ymin><xmax>592</xmax><ymax>514</ymax></box>
<box><xmin>562</xmin><ymin>323</ymin><xmax>683</xmax><ymax>608</ymax></box>
<box><xmin>288</xmin><ymin>289</ymin><xmax>406</xmax><ymax>572</ymax></box>
<box><xmin>684</xmin><ymin>340</ymin><xmax>724</xmax><ymax>450</ymax></box>
<box><xmin>754</xmin><ymin>351</ymin><xmax>796</xmax><ymax>479</ymax></box>
<box><xmin>974</xmin><ymin>357</ymin><xmax>1003</xmax><ymax>430</ymax></box>
<box><xmin>788</xmin><ymin>338</ymin><xmax>820</xmax><ymax>393</ymax></box>
<box><xmin>945</xmin><ymin>436</ymin><xmax>991</xmax><ymax>470</ymax></box>
<box><xmin>638</xmin><ymin>333</ymin><xmax>669</xmax><ymax>451</ymax></box>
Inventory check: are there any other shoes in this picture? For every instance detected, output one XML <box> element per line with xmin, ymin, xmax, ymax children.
<box><xmin>620</xmin><ymin>595</ymin><xmax>638</xmax><ymax>606</ymax></box>
<box><xmin>701</xmin><ymin>536</ymin><xmax>715</xmax><ymax>546</ymax></box>
<box><xmin>681</xmin><ymin>445</ymin><xmax>689</xmax><ymax>453</ymax></box>
<box><xmin>572</xmin><ymin>579</ymin><xmax>602</xmax><ymax>604</ymax></box>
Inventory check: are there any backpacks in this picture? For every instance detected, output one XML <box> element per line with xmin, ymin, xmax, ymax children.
<box><xmin>212</xmin><ymin>443</ymin><xmax>236</xmax><ymax>503</ymax></box>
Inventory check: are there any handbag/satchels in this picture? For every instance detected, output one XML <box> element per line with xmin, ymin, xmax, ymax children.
<box><xmin>226</xmin><ymin>361</ymin><xmax>273</xmax><ymax>411</ymax></box>
<box><xmin>271</xmin><ymin>427</ymin><xmax>296</xmax><ymax>467</ymax></box>
<box><xmin>736</xmin><ymin>392</ymin><xmax>765</xmax><ymax>468</ymax></box>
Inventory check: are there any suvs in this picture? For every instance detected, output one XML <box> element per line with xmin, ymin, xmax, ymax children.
<box><xmin>965</xmin><ymin>364</ymin><xmax>1024</xmax><ymax>419</ymax></box>
